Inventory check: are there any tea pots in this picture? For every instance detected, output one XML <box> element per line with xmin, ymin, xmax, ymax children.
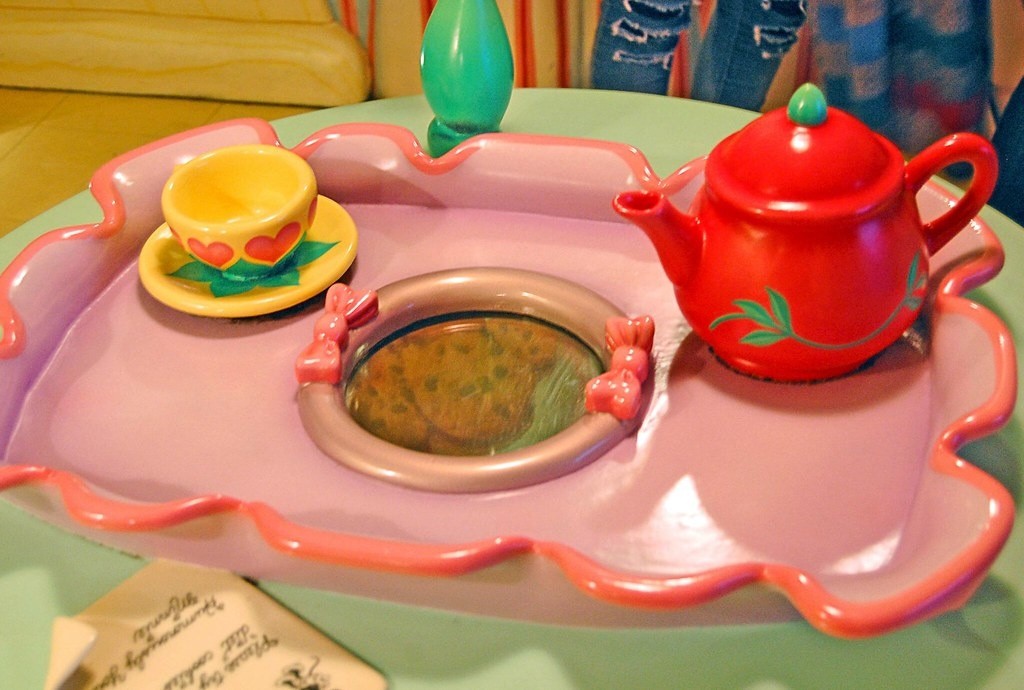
<box><xmin>611</xmin><ymin>82</ymin><xmax>999</xmax><ymax>381</ymax></box>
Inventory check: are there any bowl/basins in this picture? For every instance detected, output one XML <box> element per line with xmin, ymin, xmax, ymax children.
<box><xmin>160</xmin><ymin>145</ymin><xmax>318</xmax><ymax>284</ymax></box>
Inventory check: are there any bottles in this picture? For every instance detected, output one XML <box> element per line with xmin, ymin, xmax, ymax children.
<box><xmin>418</xmin><ymin>0</ymin><xmax>515</xmax><ymax>159</ymax></box>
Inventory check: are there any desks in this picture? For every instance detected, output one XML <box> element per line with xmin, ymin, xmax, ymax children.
<box><xmin>0</xmin><ymin>87</ymin><xmax>1024</xmax><ymax>690</ymax></box>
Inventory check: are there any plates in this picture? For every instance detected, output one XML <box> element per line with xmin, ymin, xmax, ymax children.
<box><xmin>137</xmin><ymin>195</ymin><xmax>358</xmax><ymax>318</ymax></box>
